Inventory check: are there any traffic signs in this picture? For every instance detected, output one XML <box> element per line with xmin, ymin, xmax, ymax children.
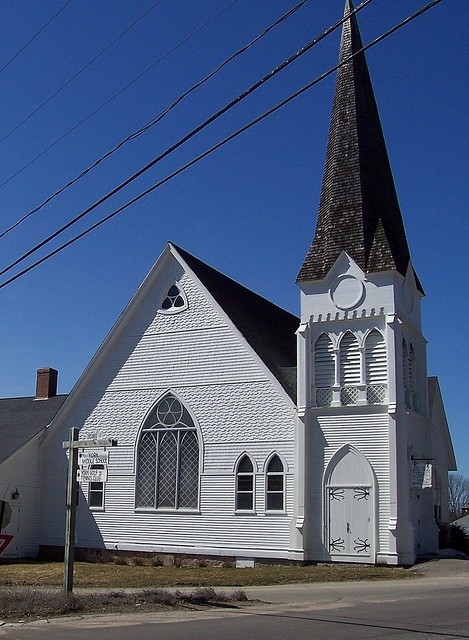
<box><xmin>77</xmin><ymin>469</ymin><xmax>107</xmax><ymax>482</ymax></box>
<box><xmin>77</xmin><ymin>451</ymin><xmax>109</xmax><ymax>464</ymax></box>
